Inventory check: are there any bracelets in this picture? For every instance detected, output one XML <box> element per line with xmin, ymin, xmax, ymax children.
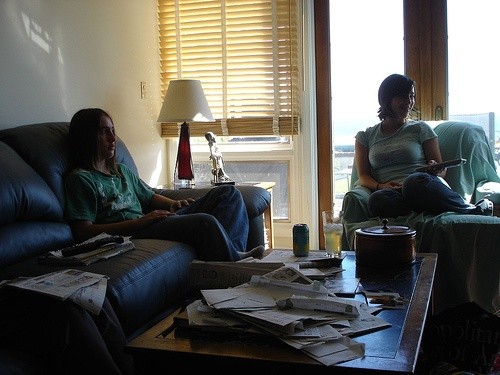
<box><xmin>377</xmin><ymin>183</ymin><xmax>380</xmax><ymax>190</ymax></box>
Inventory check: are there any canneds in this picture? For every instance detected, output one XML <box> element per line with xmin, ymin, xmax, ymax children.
<box><xmin>292</xmin><ymin>223</ymin><xmax>310</xmax><ymax>257</ymax></box>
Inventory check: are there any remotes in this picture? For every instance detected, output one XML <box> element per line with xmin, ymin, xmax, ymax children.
<box><xmin>416</xmin><ymin>158</ymin><xmax>467</xmax><ymax>172</ymax></box>
<box><xmin>62</xmin><ymin>236</ymin><xmax>125</xmax><ymax>257</ymax></box>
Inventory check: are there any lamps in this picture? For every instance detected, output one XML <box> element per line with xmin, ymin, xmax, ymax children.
<box><xmin>156</xmin><ymin>79</ymin><xmax>215</xmax><ymax>189</ymax></box>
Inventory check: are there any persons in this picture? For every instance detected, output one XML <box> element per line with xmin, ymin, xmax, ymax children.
<box><xmin>205</xmin><ymin>131</ymin><xmax>232</xmax><ymax>182</ymax></box>
<box><xmin>66</xmin><ymin>107</ymin><xmax>265</xmax><ymax>262</ymax></box>
<box><xmin>355</xmin><ymin>74</ymin><xmax>494</xmax><ymax>216</ymax></box>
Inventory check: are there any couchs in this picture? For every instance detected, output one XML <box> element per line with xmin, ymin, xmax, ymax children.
<box><xmin>338</xmin><ymin>119</ymin><xmax>500</xmax><ymax>272</ymax></box>
<box><xmin>0</xmin><ymin>121</ymin><xmax>249</xmax><ymax>334</ymax></box>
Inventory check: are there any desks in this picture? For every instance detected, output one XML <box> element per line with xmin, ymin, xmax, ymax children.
<box><xmin>114</xmin><ymin>251</ymin><xmax>436</xmax><ymax>375</ymax></box>
<box><xmin>157</xmin><ymin>182</ymin><xmax>277</xmax><ymax>248</ymax></box>
